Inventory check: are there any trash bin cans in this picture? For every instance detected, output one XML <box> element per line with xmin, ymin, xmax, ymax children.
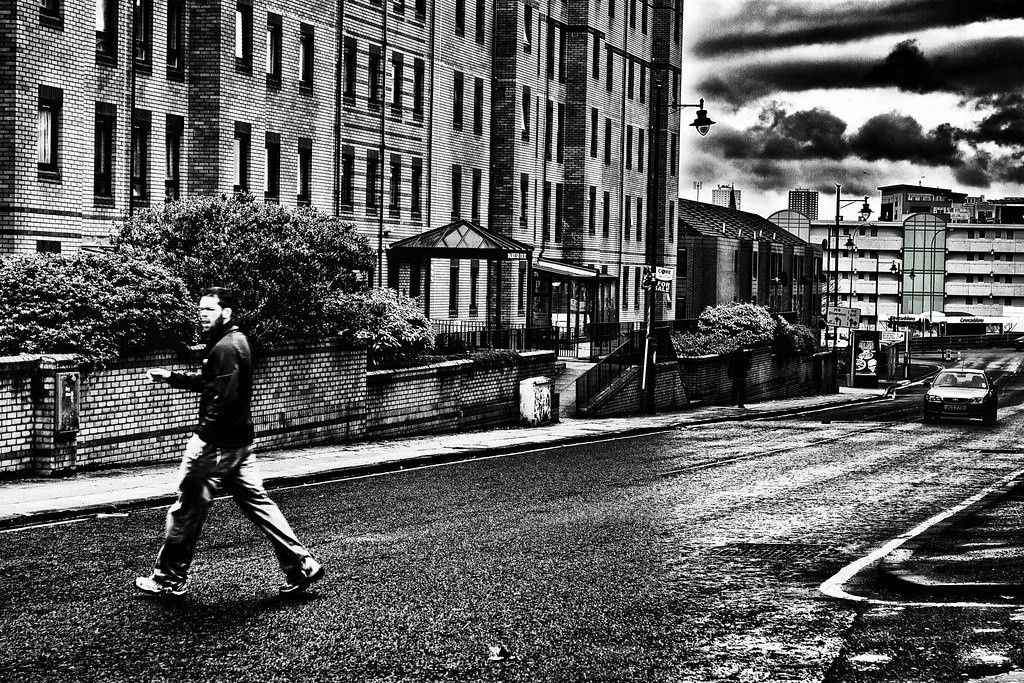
<box><xmin>519</xmin><ymin>376</ymin><xmax>551</xmax><ymax>427</ymax></box>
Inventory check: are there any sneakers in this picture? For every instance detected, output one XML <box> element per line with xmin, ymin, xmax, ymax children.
<box><xmin>132</xmin><ymin>566</ymin><xmax>192</xmax><ymax>598</ymax></box>
<box><xmin>279</xmin><ymin>556</ymin><xmax>327</xmax><ymax>597</ymax></box>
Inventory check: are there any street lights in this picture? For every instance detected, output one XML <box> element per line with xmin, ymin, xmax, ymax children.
<box><xmin>825</xmin><ymin>229</ymin><xmax>857</xmax><ymax>357</ymax></box>
<box><xmin>645</xmin><ymin>78</ymin><xmax>716</xmax><ymax>417</ymax></box>
<box><xmin>888</xmin><ymin>260</ymin><xmax>900</xmax><ymax>364</ymax></box>
<box><xmin>829</xmin><ymin>181</ymin><xmax>876</xmax><ymax>386</ymax></box>
<box><xmin>929</xmin><ymin>227</ymin><xmax>954</xmax><ymax>338</ymax></box>
<box><xmin>847</xmin><ymin>222</ymin><xmax>875</xmax><ymax>348</ymax></box>
<box><xmin>909</xmin><ymin>268</ymin><xmax>916</xmax><ymax>315</ymax></box>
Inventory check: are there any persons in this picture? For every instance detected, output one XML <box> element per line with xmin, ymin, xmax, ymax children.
<box><xmin>137</xmin><ymin>286</ymin><xmax>325</xmax><ymax>597</ymax></box>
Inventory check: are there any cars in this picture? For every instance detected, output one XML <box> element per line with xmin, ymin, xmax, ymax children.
<box><xmin>922</xmin><ymin>369</ymin><xmax>1000</xmax><ymax>426</ymax></box>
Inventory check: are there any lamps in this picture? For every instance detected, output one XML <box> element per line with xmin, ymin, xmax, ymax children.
<box><xmin>652</xmin><ymin>97</ymin><xmax>717</xmax><ymax>136</ymax></box>
